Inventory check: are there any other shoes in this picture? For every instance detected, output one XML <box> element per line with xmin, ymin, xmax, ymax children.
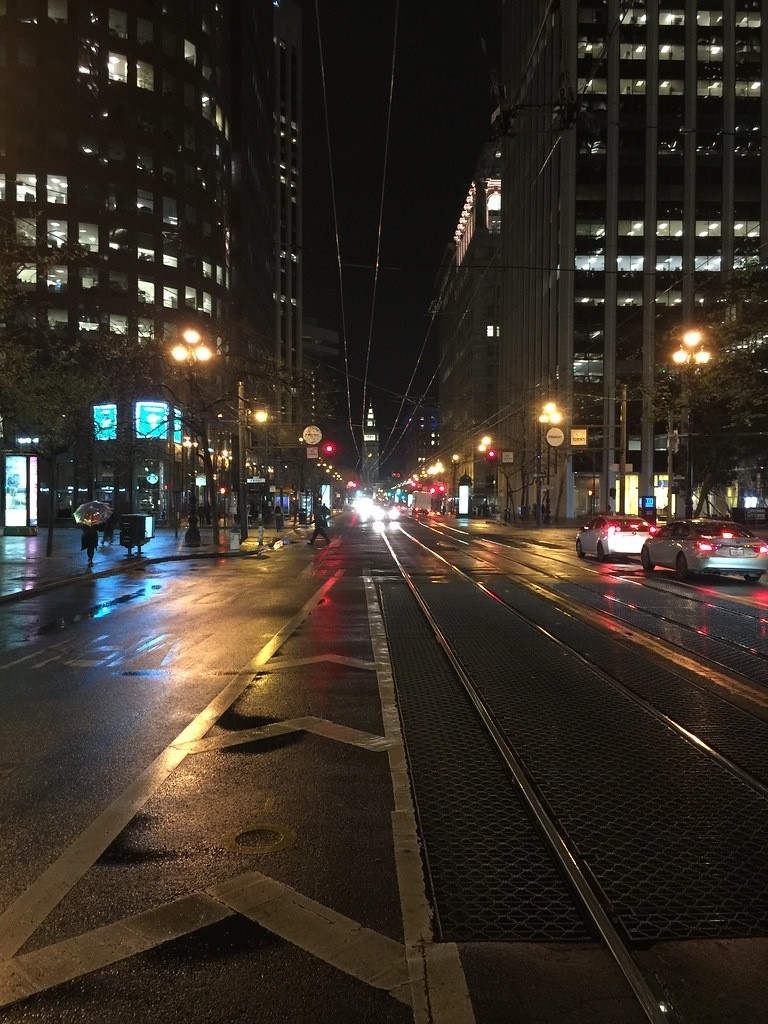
<box><xmin>326</xmin><ymin>540</ymin><xmax>331</xmax><ymax>545</ymax></box>
<box><xmin>307</xmin><ymin>540</ymin><xmax>314</xmax><ymax>544</ymax></box>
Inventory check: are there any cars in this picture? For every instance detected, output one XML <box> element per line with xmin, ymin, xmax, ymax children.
<box><xmin>374</xmin><ymin>506</ymin><xmax>397</xmax><ymax>520</ymax></box>
<box><xmin>640</xmin><ymin>518</ymin><xmax>768</xmax><ymax>583</ymax></box>
<box><xmin>576</xmin><ymin>514</ymin><xmax>658</xmax><ymax>563</ymax></box>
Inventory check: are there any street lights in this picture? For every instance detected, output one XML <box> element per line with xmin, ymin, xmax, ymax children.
<box><xmin>169</xmin><ymin>328</ymin><xmax>212</xmax><ymax>548</ymax></box>
<box><xmin>671</xmin><ymin>329</ymin><xmax>711</xmax><ymax>518</ymax></box>
<box><xmin>480</xmin><ymin>435</ymin><xmax>491</xmax><ymax>515</ymax></box>
<box><xmin>538</xmin><ymin>401</ymin><xmax>562</xmax><ymax>525</ymax></box>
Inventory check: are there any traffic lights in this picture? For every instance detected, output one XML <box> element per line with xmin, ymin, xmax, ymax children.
<box><xmin>324</xmin><ymin>437</ymin><xmax>334</xmax><ymax>454</ymax></box>
<box><xmin>430</xmin><ymin>487</ymin><xmax>436</xmax><ymax>493</ymax></box>
<box><xmin>487</xmin><ymin>448</ymin><xmax>495</xmax><ymax>459</ymax></box>
<box><xmin>440</xmin><ymin>485</ymin><xmax>445</xmax><ymax>493</ymax></box>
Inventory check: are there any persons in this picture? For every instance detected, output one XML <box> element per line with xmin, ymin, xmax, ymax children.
<box><xmin>306</xmin><ymin>495</ymin><xmax>331</xmax><ymax>545</ymax></box>
<box><xmin>205</xmin><ymin>502</ymin><xmax>212</xmax><ymax>525</ymax></box>
<box><xmin>100</xmin><ymin>502</ymin><xmax>113</xmax><ymax>544</ymax></box>
<box><xmin>80</xmin><ymin>525</ymin><xmax>108</xmax><ymax>566</ymax></box>
<box><xmin>198</xmin><ymin>502</ymin><xmax>205</xmax><ymax>526</ymax></box>
<box><xmin>292</xmin><ymin>503</ymin><xmax>297</xmax><ymax>526</ymax></box>
<box><xmin>274</xmin><ymin>502</ymin><xmax>282</xmax><ymax>532</ymax></box>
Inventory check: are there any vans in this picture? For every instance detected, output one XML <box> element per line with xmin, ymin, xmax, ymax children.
<box><xmin>442</xmin><ymin>498</ymin><xmax>459</xmax><ymax>512</ymax></box>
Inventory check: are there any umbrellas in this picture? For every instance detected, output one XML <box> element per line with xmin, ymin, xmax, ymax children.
<box><xmin>73</xmin><ymin>500</ymin><xmax>114</xmax><ymax>527</ymax></box>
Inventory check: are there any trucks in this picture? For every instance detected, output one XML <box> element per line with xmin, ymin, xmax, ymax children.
<box><xmin>414</xmin><ymin>492</ymin><xmax>432</xmax><ymax>514</ymax></box>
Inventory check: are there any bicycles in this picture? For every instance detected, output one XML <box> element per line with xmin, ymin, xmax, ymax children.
<box><xmin>274</xmin><ymin>513</ymin><xmax>283</xmax><ymax>531</ymax></box>
